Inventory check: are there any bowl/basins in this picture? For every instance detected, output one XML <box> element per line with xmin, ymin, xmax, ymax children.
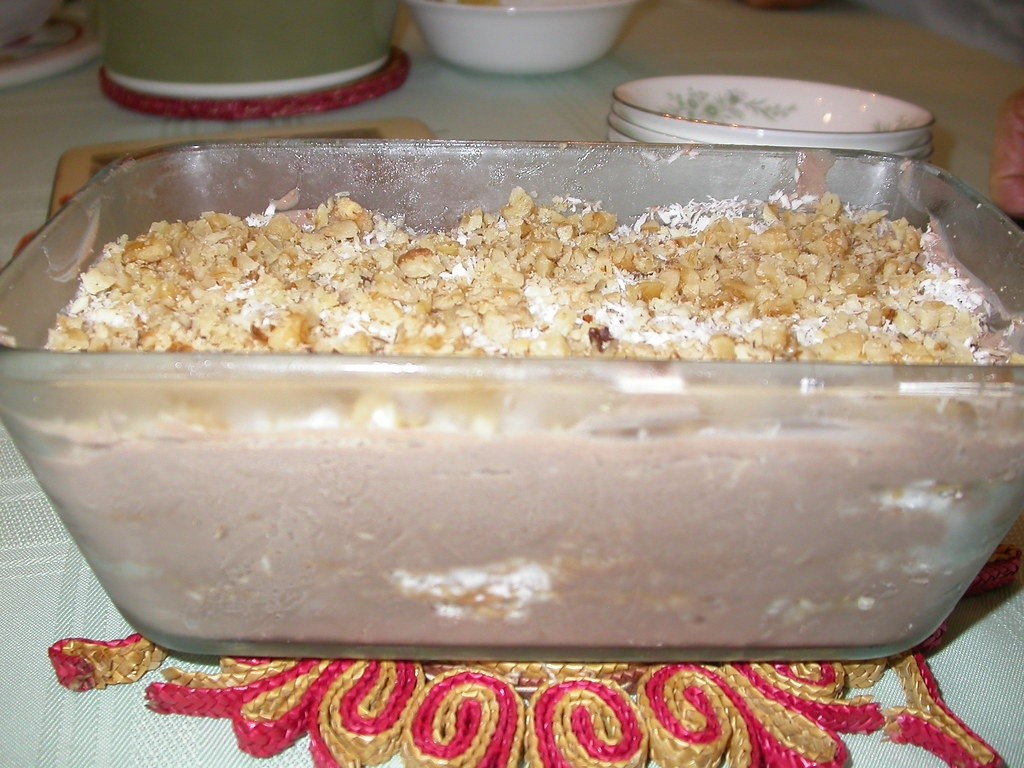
<box><xmin>0</xmin><ymin>138</ymin><xmax>1024</xmax><ymax>662</ymax></box>
<box><xmin>411</xmin><ymin>0</ymin><xmax>633</xmax><ymax>74</ymax></box>
<box><xmin>608</xmin><ymin>75</ymin><xmax>937</xmax><ymax>167</ymax></box>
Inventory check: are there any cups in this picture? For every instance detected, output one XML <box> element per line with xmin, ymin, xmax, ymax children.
<box><xmin>101</xmin><ymin>0</ymin><xmax>400</xmax><ymax>98</ymax></box>
